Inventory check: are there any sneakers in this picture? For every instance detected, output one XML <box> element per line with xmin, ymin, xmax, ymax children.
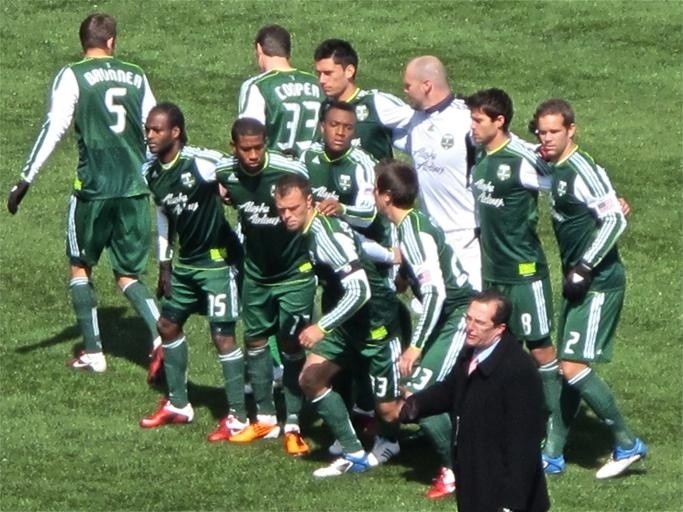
<box><xmin>244</xmin><ymin>368</ymin><xmax>285</xmax><ymax>393</ymax></box>
<box><xmin>596</xmin><ymin>438</ymin><xmax>646</xmax><ymax>480</ymax></box>
<box><xmin>66</xmin><ymin>350</ymin><xmax>107</xmax><ymax>373</ymax></box>
<box><xmin>313</xmin><ymin>449</ymin><xmax>378</xmax><ymax>477</ymax></box>
<box><xmin>228</xmin><ymin>415</ymin><xmax>280</xmax><ymax>444</ymax></box>
<box><xmin>147</xmin><ymin>344</ymin><xmax>164</xmax><ymax>383</ymax></box>
<box><xmin>330</xmin><ymin>440</ymin><xmax>344</xmax><ymax>454</ymax></box>
<box><xmin>541</xmin><ymin>453</ymin><xmax>566</xmax><ymax>474</ymax></box>
<box><xmin>209</xmin><ymin>416</ymin><xmax>250</xmax><ymax>441</ymax></box>
<box><xmin>284</xmin><ymin>424</ymin><xmax>310</xmax><ymax>455</ymax></box>
<box><xmin>369</xmin><ymin>435</ymin><xmax>400</xmax><ymax>466</ymax></box>
<box><xmin>140</xmin><ymin>398</ymin><xmax>193</xmax><ymax>427</ymax></box>
<box><xmin>425</xmin><ymin>466</ymin><xmax>456</xmax><ymax>499</ymax></box>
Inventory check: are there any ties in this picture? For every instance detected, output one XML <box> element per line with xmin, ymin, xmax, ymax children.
<box><xmin>468</xmin><ymin>356</ymin><xmax>478</xmax><ymax>377</ymax></box>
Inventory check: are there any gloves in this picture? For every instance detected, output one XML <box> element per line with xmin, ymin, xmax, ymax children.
<box><xmin>156</xmin><ymin>260</ymin><xmax>172</xmax><ymax>301</ymax></box>
<box><xmin>8</xmin><ymin>180</ymin><xmax>31</xmax><ymax>215</ymax></box>
<box><xmin>562</xmin><ymin>261</ymin><xmax>593</xmax><ymax>304</ymax></box>
<box><xmin>400</xmin><ymin>394</ymin><xmax>419</xmax><ymax>424</ymax></box>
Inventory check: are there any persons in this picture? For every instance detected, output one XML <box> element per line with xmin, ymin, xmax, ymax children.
<box><xmin>398</xmin><ymin>289</ymin><xmax>552</xmax><ymax>512</ymax></box>
<box><xmin>466</xmin><ymin>88</ymin><xmax>631</xmax><ymax>475</ymax></box>
<box><xmin>141</xmin><ymin>103</ymin><xmax>249</xmax><ymax>443</ymax></box>
<box><xmin>311</xmin><ymin>38</ymin><xmax>413</xmax><ymax>163</ymax></box>
<box><xmin>7</xmin><ymin>15</ymin><xmax>163</xmax><ymax>386</ymax></box>
<box><xmin>533</xmin><ymin>100</ymin><xmax>648</xmax><ymax>480</ymax></box>
<box><xmin>215</xmin><ymin>118</ymin><xmax>316</xmax><ymax>456</ymax></box>
<box><xmin>373</xmin><ymin>160</ymin><xmax>478</xmax><ymax>499</ymax></box>
<box><xmin>298</xmin><ymin>101</ymin><xmax>382</xmax><ymax>228</ymax></box>
<box><xmin>275</xmin><ymin>173</ymin><xmax>401</xmax><ymax>478</ymax></box>
<box><xmin>403</xmin><ymin>56</ymin><xmax>541</xmax><ymax>291</ymax></box>
<box><xmin>237</xmin><ymin>25</ymin><xmax>325</xmax><ymax>163</ymax></box>
